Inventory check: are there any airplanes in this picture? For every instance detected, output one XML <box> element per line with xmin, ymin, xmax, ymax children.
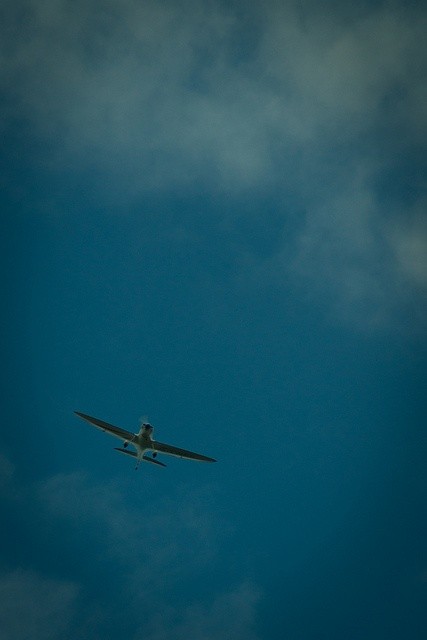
<box><xmin>73</xmin><ymin>412</ymin><xmax>219</xmax><ymax>472</ymax></box>
